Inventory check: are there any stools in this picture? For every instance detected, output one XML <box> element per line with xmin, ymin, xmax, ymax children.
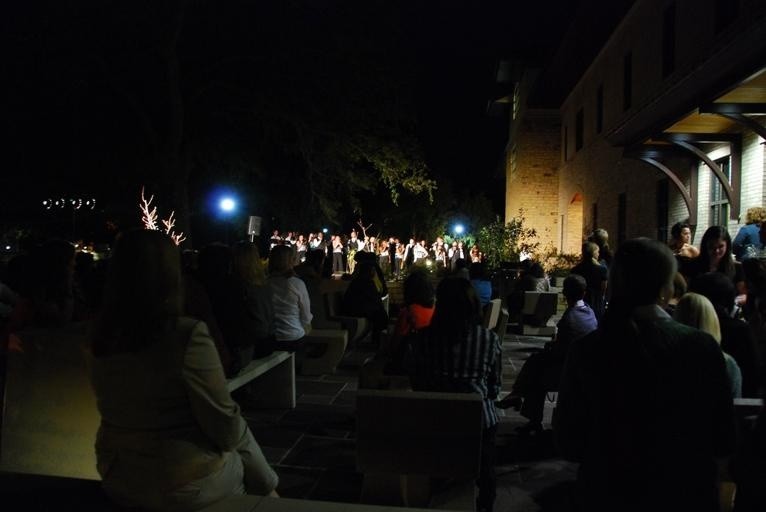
<box><xmin>296</xmin><ymin>326</ymin><xmax>348</xmax><ymax>376</ymax></box>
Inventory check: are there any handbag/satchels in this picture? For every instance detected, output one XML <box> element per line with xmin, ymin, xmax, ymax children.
<box><xmin>594</xmin><ymin>295</ymin><xmax>606</xmax><ymax>319</ymax></box>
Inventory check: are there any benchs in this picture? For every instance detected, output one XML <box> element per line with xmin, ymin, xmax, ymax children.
<box><xmin>225</xmin><ymin>350</ymin><xmax>296</xmax><ymax>412</ymax></box>
<box><xmin>0</xmin><ymin>471</ymin><xmax>456</xmax><ymax>512</ymax></box>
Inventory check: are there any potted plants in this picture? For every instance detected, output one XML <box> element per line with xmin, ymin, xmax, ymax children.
<box><xmin>551</xmin><ymin>267</ymin><xmax>569</xmax><ymax>286</ymax></box>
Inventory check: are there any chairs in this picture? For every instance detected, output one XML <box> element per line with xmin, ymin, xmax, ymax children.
<box><xmin>354</xmin><ymin>385</ymin><xmax>483</xmax><ymax>506</ymax></box>
<box><xmin>490</xmin><ymin>308</ymin><xmax>510</xmax><ymax>348</ymax></box>
<box><xmin>322</xmin><ymin>290</ymin><xmax>372</xmax><ymax>350</ymax></box>
<box><xmin>518</xmin><ymin>289</ymin><xmax>558</xmax><ymax>335</ymax></box>
<box><xmin>484</xmin><ymin>298</ymin><xmax>502</xmax><ymax>330</ymax></box>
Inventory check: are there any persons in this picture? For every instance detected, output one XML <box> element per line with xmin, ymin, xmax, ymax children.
<box><xmin>410</xmin><ymin>277</ymin><xmax>503</xmax><ymax>512</ymax></box>
<box><xmin>293</xmin><ymin>249</ymin><xmax>391</xmax><ymax>343</ymax></box>
<box><xmin>552</xmin><ymin>237</ymin><xmax>731</xmax><ymax>512</ymax></box>
<box><xmin>270</xmin><ymin>229</ymin><xmax>483</xmax><ymax>281</ymax></box>
<box><xmin>91</xmin><ymin>228</ymin><xmax>279</xmax><ymax>511</ymax></box>
<box><xmin>397</xmin><ymin>207</ymin><xmax>765</xmax><ymax>434</ymax></box>
<box><xmin>186</xmin><ymin>241</ymin><xmax>313</xmax><ymax>376</ymax></box>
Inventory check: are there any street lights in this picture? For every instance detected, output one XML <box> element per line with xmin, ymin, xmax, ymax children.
<box><xmin>322</xmin><ymin>228</ymin><xmax>328</xmax><ymax>236</ymax></box>
<box><xmin>218</xmin><ymin>197</ymin><xmax>239</xmax><ymax>245</ymax></box>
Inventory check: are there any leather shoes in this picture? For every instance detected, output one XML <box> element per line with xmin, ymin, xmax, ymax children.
<box><xmin>514</xmin><ymin>421</ymin><xmax>543</xmax><ymax>433</ymax></box>
<box><xmin>494</xmin><ymin>396</ymin><xmax>522</xmax><ymax>411</ymax></box>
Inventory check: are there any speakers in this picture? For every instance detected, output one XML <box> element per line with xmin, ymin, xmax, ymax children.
<box><xmin>248</xmin><ymin>215</ymin><xmax>263</xmax><ymax>236</ymax></box>
